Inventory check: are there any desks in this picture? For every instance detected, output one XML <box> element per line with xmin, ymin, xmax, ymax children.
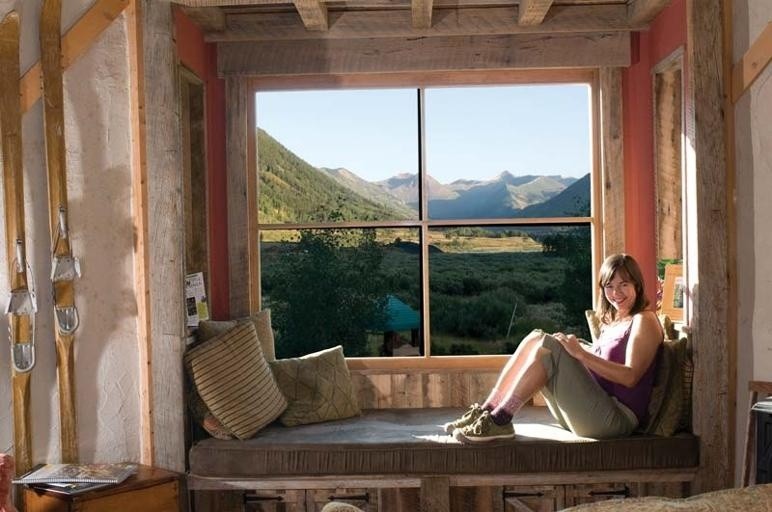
<box><xmin>17</xmin><ymin>461</ymin><xmax>188</xmax><ymax>512</ymax></box>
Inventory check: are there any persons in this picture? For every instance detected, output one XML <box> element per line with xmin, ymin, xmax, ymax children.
<box><xmin>442</xmin><ymin>252</ymin><xmax>665</xmax><ymax>445</ymax></box>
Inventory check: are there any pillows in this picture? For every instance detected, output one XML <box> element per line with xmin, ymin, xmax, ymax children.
<box><xmin>584</xmin><ymin>309</ymin><xmax>694</xmax><ymax>439</ymax></box>
<box><xmin>183</xmin><ymin>307</ymin><xmax>361</xmax><ymax>442</ymax></box>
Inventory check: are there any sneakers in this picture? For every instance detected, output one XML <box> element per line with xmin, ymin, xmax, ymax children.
<box><xmin>443</xmin><ymin>404</ymin><xmax>515</xmax><ymax>445</ymax></box>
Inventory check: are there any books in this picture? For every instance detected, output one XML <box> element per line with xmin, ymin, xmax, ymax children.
<box><xmin>11</xmin><ymin>461</ymin><xmax>137</xmax><ymax>486</ymax></box>
<box><xmin>25</xmin><ymin>471</ymin><xmax>137</xmax><ymax>496</ymax></box>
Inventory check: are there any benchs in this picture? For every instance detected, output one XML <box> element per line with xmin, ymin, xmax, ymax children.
<box><xmin>185</xmin><ymin>409</ymin><xmax>701</xmax><ymax>512</ymax></box>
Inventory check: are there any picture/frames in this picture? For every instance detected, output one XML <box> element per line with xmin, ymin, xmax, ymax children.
<box><xmin>660</xmin><ymin>262</ymin><xmax>684</xmax><ymax>323</ymax></box>
